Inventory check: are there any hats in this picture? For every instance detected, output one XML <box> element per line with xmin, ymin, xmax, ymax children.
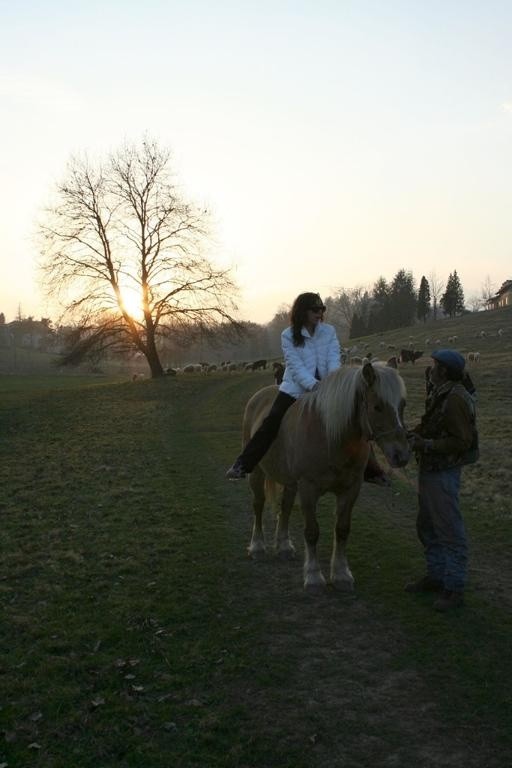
<box><xmin>431</xmin><ymin>349</ymin><xmax>465</xmax><ymax>372</ymax></box>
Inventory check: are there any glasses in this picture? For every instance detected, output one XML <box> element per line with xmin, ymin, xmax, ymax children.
<box><xmin>309</xmin><ymin>305</ymin><xmax>326</xmax><ymax>314</ymax></box>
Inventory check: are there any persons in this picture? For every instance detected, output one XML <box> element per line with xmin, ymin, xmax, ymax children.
<box><xmin>225</xmin><ymin>291</ymin><xmax>396</xmax><ymax>487</ymax></box>
<box><xmin>402</xmin><ymin>350</ymin><xmax>491</xmax><ymax>611</ymax></box>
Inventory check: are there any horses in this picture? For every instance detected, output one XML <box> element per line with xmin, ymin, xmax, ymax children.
<box><xmin>241</xmin><ymin>357</ymin><xmax>412</xmax><ymax>596</ymax></box>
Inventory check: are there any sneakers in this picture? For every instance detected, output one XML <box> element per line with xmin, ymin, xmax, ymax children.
<box><xmin>365</xmin><ymin>464</ymin><xmax>394</xmax><ymax>487</ymax></box>
<box><xmin>403</xmin><ymin>573</ymin><xmax>444</xmax><ymax>593</ymax></box>
<box><xmin>225</xmin><ymin>457</ymin><xmax>253</xmax><ymax>480</ymax></box>
<box><xmin>433</xmin><ymin>592</ymin><xmax>461</xmax><ymax>613</ymax></box>
